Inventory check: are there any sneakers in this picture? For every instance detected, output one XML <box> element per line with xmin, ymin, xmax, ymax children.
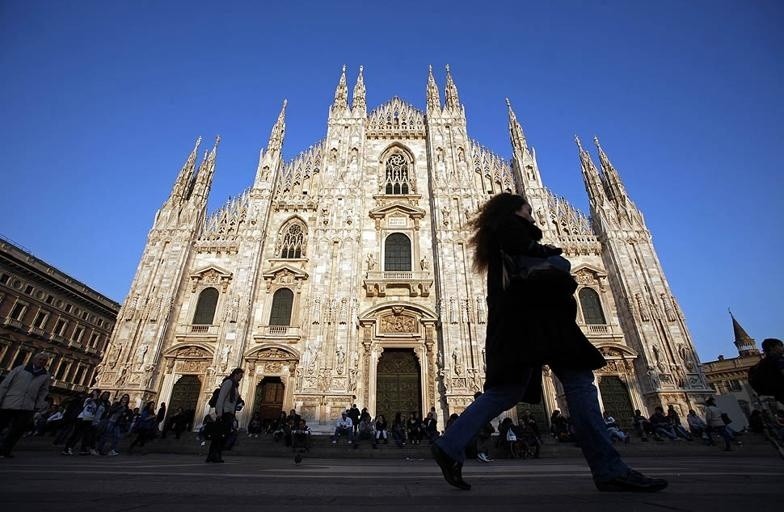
<box><xmin>478</xmin><ymin>453</ymin><xmax>488</xmax><ymax>462</ymax></box>
<box><xmin>594</xmin><ymin>469</ymin><xmax>668</xmax><ymax>492</ymax></box>
<box><xmin>431</xmin><ymin>443</ymin><xmax>471</xmax><ymax>490</ymax></box>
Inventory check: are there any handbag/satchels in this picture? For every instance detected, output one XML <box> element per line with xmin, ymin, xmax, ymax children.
<box><xmin>501</xmin><ymin>252</ymin><xmax>571</xmax><ymax>312</ymax></box>
<box><xmin>209</xmin><ymin>389</ymin><xmax>221</xmax><ymax>408</ymax></box>
<box><xmin>204</xmin><ymin>421</ymin><xmax>228</xmax><ymax>441</ymax></box>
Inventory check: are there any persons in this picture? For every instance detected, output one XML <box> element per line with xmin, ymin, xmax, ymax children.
<box><xmin>207</xmin><ymin>367</ymin><xmax>244</xmax><ymax>464</ymax></box>
<box><xmin>2</xmin><ymin>353</ymin><xmax>51</xmax><ymax>457</ymax></box>
<box><xmin>429</xmin><ymin>195</ymin><xmax>669</xmax><ymax>491</ymax></box>
<box><xmin>24</xmin><ymin>339</ymin><xmax>783</xmax><ymax>467</ymax></box>
